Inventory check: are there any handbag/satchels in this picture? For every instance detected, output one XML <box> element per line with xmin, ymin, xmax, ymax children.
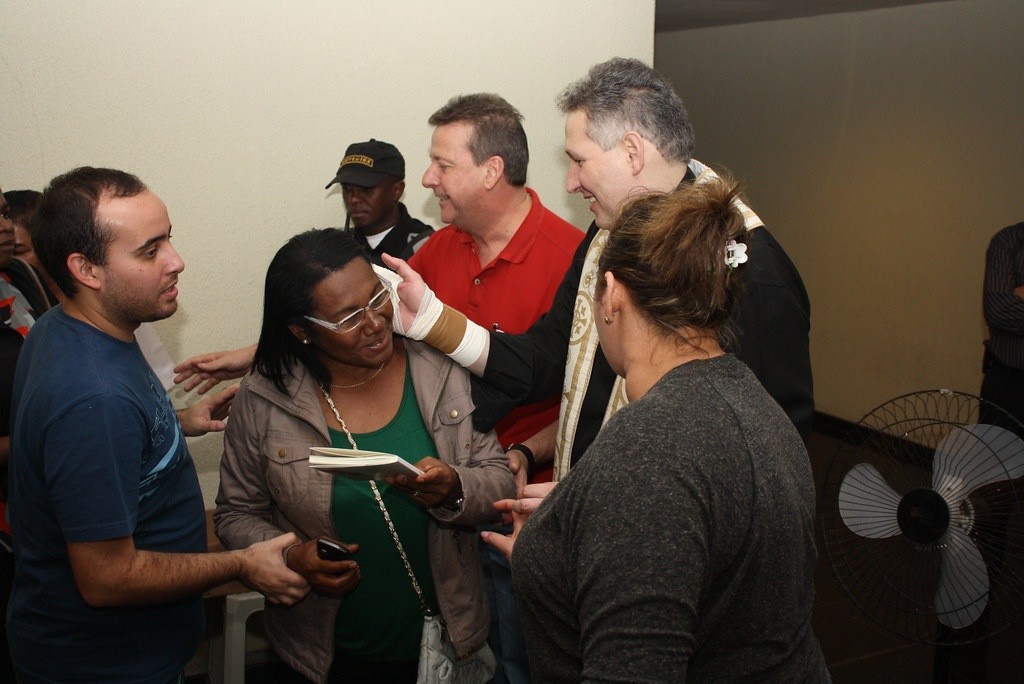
<box><xmin>415</xmin><ymin>614</ymin><xmax>497</xmax><ymax>684</ymax></box>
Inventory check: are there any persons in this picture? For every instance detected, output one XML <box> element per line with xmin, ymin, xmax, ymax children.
<box><xmin>979</xmin><ymin>222</ymin><xmax>1024</xmax><ymax>524</ymax></box>
<box><xmin>0</xmin><ymin>166</ymin><xmax>310</xmax><ymax>684</ymax></box>
<box><xmin>211</xmin><ymin>227</ymin><xmax>515</xmax><ymax>684</ymax></box>
<box><xmin>325</xmin><ymin>139</ymin><xmax>435</xmax><ymax>274</ymax></box>
<box><xmin>370</xmin><ymin>57</ymin><xmax>816</xmax><ymax>511</ymax></box>
<box><xmin>480</xmin><ymin>181</ymin><xmax>833</xmax><ymax>684</ymax></box>
<box><xmin>173</xmin><ymin>93</ymin><xmax>586</xmax><ymax>684</ymax></box>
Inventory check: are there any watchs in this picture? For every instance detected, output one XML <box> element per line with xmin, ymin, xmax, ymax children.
<box><xmin>444</xmin><ymin>498</ymin><xmax>463</xmax><ymax>510</ymax></box>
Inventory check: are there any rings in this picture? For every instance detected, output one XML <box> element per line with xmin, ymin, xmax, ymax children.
<box><xmin>413</xmin><ymin>489</ymin><xmax>420</xmax><ymax>496</ymax></box>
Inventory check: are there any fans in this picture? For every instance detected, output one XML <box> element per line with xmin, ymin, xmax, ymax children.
<box><xmin>822</xmin><ymin>388</ymin><xmax>1023</xmax><ymax>646</ymax></box>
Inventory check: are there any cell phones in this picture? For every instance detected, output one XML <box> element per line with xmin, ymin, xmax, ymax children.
<box><xmin>316</xmin><ymin>538</ymin><xmax>351</xmax><ymax>576</ymax></box>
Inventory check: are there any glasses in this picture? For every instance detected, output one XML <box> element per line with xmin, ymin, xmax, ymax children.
<box><xmin>304</xmin><ymin>272</ymin><xmax>393</xmax><ymax>334</ymax></box>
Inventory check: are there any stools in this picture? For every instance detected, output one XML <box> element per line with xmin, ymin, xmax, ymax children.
<box><xmin>209</xmin><ymin>591</ymin><xmax>265</xmax><ymax>684</ymax></box>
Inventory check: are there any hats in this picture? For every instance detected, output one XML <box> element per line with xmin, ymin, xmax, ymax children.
<box><xmin>326</xmin><ymin>139</ymin><xmax>405</xmax><ymax>188</ymax></box>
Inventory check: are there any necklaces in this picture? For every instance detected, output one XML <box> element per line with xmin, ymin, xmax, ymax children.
<box><xmin>331</xmin><ymin>363</ymin><xmax>385</xmax><ymax>388</ymax></box>
